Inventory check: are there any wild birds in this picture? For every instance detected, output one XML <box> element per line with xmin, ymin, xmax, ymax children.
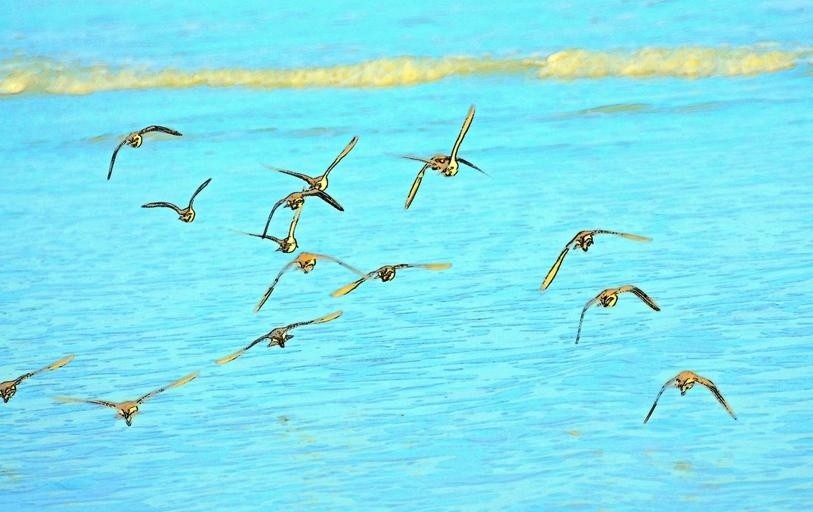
<box><xmin>212</xmin><ymin>310</ymin><xmax>343</xmax><ymax>365</ymax></box>
<box><xmin>261</xmin><ymin>135</ymin><xmax>360</xmax><ymax>190</ymax></box>
<box><xmin>233</xmin><ymin>207</ymin><xmax>305</xmax><ymax>254</ymax></box>
<box><xmin>398</xmin><ymin>104</ymin><xmax>496</xmax><ymax>208</ymax></box>
<box><xmin>260</xmin><ymin>190</ymin><xmax>344</xmax><ymax>238</ymax></box>
<box><xmin>642</xmin><ymin>370</ymin><xmax>739</xmax><ymax>425</ymax></box>
<box><xmin>574</xmin><ymin>285</ymin><xmax>661</xmax><ymax>345</ymax></box>
<box><xmin>253</xmin><ymin>252</ymin><xmax>368</xmax><ymax>312</ymax></box>
<box><xmin>539</xmin><ymin>229</ymin><xmax>652</xmax><ymax>291</ymax></box>
<box><xmin>330</xmin><ymin>262</ymin><xmax>454</xmax><ymax>297</ymax></box>
<box><xmin>53</xmin><ymin>372</ymin><xmax>199</xmax><ymax>427</ymax></box>
<box><xmin>1</xmin><ymin>356</ymin><xmax>75</xmax><ymax>405</ymax></box>
<box><xmin>141</xmin><ymin>178</ymin><xmax>213</xmax><ymax>224</ymax></box>
<box><xmin>107</xmin><ymin>124</ymin><xmax>184</xmax><ymax>181</ymax></box>
